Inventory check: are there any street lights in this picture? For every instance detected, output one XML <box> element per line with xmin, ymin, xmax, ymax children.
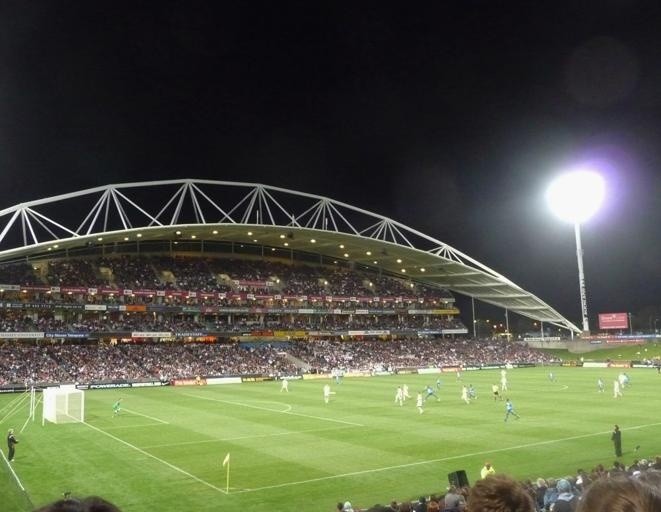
<box><xmin>544</xmin><ymin>166</ymin><xmax>613</xmax><ymax>340</ymax></box>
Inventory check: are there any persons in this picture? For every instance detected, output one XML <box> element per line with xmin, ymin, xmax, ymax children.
<box><xmin>547</xmin><ymin>370</ymin><xmax>554</xmax><ymax>383</ymax></box>
<box><xmin>322</xmin><ymin>384</ymin><xmax>331</xmax><ymax>404</ymax></box>
<box><xmin>617</xmin><ymin>372</ymin><xmax>628</xmax><ymax>389</ymax></box>
<box><xmin>471</xmin><ymin>330</ymin><xmax>661</xmax><ymax>368</ymax></box>
<box><xmin>279</xmin><ymin>379</ymin><xmax>288</xmax><ymax>392</ymax></box>
<box><xmin>393</xmin><ymin>368</ymin><xmax>509</xmax><ymax>415</ymax></box>
<box><xmin>111</xmin><ymin>397</ymin><xmax>123</xmax><ymax>419</ymax></box>
<box><xmin>333</xmin><ymin>453</ymin><xmax>660</xmax><ymax>511</ymax></box>
<box><xmin>610</xmin><ymin>424</ymin><xmax>623</xmax><ymax>458</ymax></box>
<box><xmin>612</xmin><ymin>378</ymin><xmax>622</xmax><ymax>399</ymax></box>
<box><xmin>596</xmin><ymin>376</ymin><xmax>605</xmax><ymax>393</ymax></box>
<box><xmin>622</xmin><ymin>372</ymin><xmax>631</xmax><ymax>388</ymax></box>
<box><xmin>1</xmin><ymin>249</ymin><xmax>471</xmax><ymax>385</ymax></box>
<box><xmin>6</xmin><ymin>427</ymin><xmax>18</xmax><ymax>465</ymax></box>
<box><xmin>503</xmin><ymin>398</ymin><xmax>520</xmax><ymax>423</ymax></box>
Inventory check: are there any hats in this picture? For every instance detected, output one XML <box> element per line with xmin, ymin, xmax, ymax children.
<box><xmin>558</xmin><ymin>480</ymin><xmax>570</xmax><ymax>491</ymax></box>
<box><xmin>633</xmin><ymin>471</ymin><xmax>641</xmax><ymax>477</ymax></box>
<box><xmin>640</xmin><ymin>459</ymin><xmax>647</xmax><ymax>465</ymax></box>
<box><xmin>576</xmin><ymin>479</ymin><xmax>583</xmax><ymax>484</ymax></box>
<box><xmin>418</xmin><ymin>497</ymin><xmax>426</xmax><ymax>503</ymax></box>
